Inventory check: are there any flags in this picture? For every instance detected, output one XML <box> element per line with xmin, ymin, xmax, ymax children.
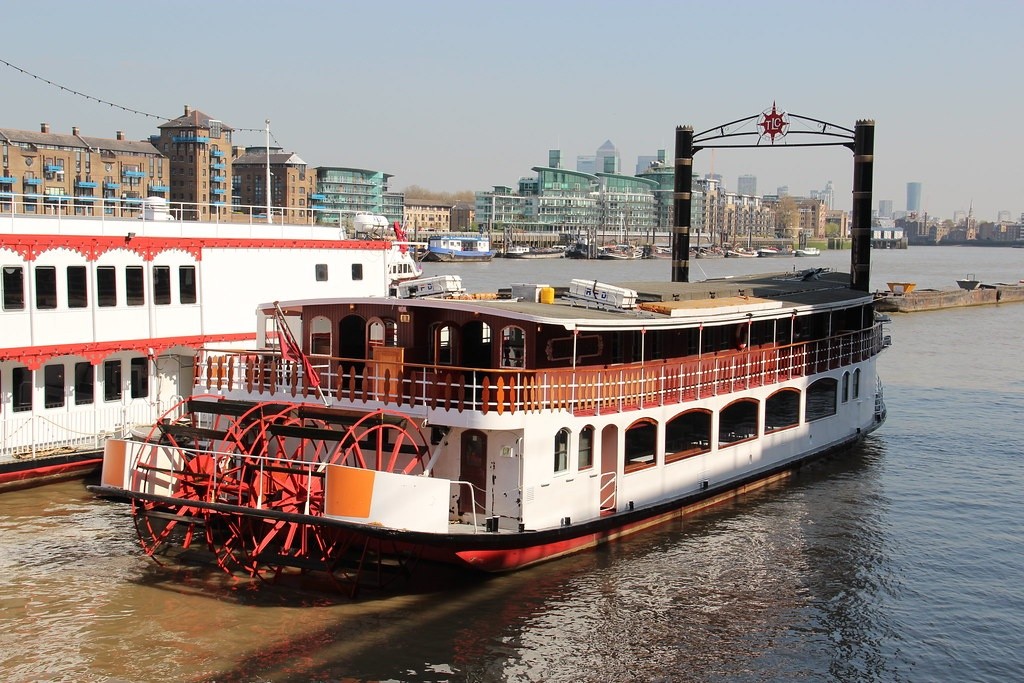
<box><xmin>276</xmin><ymin>311</ymin><xmax>320</xmax><ymax>388</ymax></box>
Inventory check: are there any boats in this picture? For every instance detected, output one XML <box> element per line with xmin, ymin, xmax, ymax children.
<box><xmin>421</xmin><ymin>227</ymin><xmax>498</xmax><ymax>262</ymax></box>
<box><xmin>795</xmin><ymin>247</ymin><xmax>820</xmax><ymax>257</ymax></box>
<box><xmin>758</xmin><ymin>236</ymin><xmax>797</xmax><ymax>258</ymax></box>
<box><xmin>644</xmin><ymin>228</ymin><xmax>697</xmax><ymax>260</ymax></box>
<box><xmin>0</xmin><ymin>118</ymin><xmax>392</xmax><ymax>494</ymax></box>
<box><xmin>565</xmin><ymin>224</ymin><xmax>643</xmax><ymax>260</ymax></box>
<box><xmin>726</xmin><ymin>230</ymin><xmax>760</xmax><ymax>258</ymax></box>
<box><xmin>872</xmin><ymin>274</ymin><xmax>1024</xmax><ymax>312</ymax></box>
<box><xmin>495</xmin><ymin>228</ymin><xmax>567</xmax><ymax>259</ymax></box>
<box><xmin>696</xmin><ymin>227</ymin><xmax>727</xmax><ymax>260</ymax></box>
<box><xmin>390</xmin><ymin>245</ymin><xmax>424</xmax><ymax>288</ymax></box>
<box><xmin>86</xmin><ymin>267</ymin><xmax>893</xmax><ymax>571</ymax></box>
